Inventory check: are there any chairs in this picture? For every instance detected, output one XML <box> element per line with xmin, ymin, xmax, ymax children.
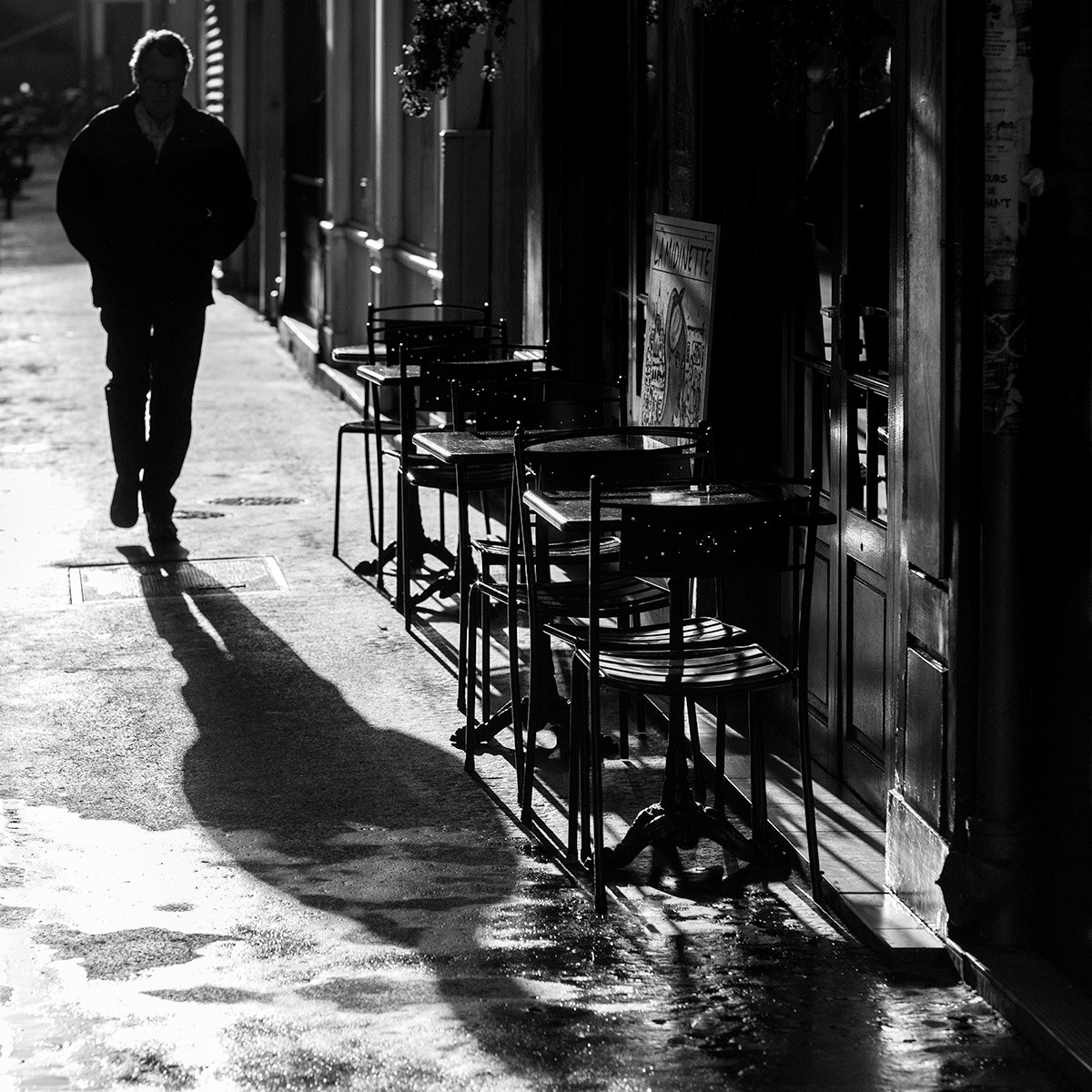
<box><xmin>327</xmin><ymin>295</ymin><xmax>825</xmax><ymax>915</ymax></box>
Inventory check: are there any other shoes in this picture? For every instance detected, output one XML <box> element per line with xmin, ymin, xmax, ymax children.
<box><xmin>110</xmin><ymin>471</ymin><xmax>140</xmax><ymax>527</ymax></box>
<box><xmin>145</xmin><ymin>509</ymin><xmax>178</xmax><ymax>539</ymax></box>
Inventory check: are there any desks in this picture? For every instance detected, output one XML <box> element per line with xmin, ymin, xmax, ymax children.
<box><xmin>519</xmin><ymin>479</ymin><xmax>836</xmax><ymax>874</ymax></box>
<box><xmin>331</xmin><ymin>340</ymin><xmax>539</xmax><ymax>576</ymax></box>
<box><xmin>353</xmin><ymin>350</ymin><xmax>564</xmax><ymax>606</ymax></box>
<box><xmin>408</xmin><ymin>420</ymin><xmax>711</xmax><ymax>761</ymax></box>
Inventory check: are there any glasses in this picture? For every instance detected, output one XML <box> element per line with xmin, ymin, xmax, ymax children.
<box><xmin>137</xmin><ymin>67</ymin><xmax>184</xmax><ymax>90</ymax></box>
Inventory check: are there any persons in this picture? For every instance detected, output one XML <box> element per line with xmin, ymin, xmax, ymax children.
<box><xmin>56</xmin><ymin>28</ymin><xmax>260</xmax><ymax>544</ymax></box>
